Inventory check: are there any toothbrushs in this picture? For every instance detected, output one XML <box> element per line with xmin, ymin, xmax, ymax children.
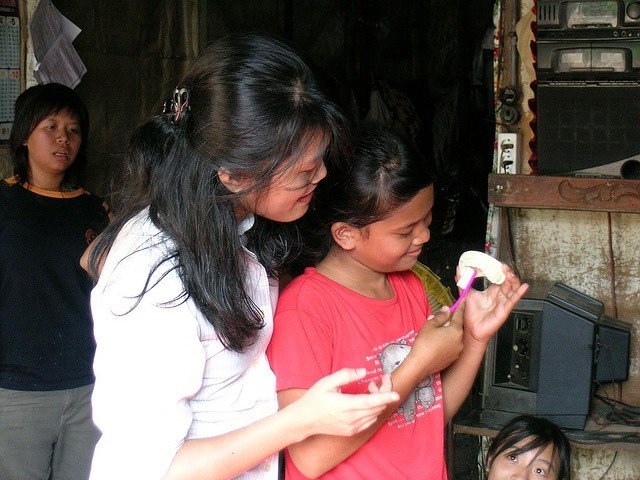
<box><xmin>438</xmin><ymin>264</ymin><xmax>480</xmax><ymax>316</ymax></box>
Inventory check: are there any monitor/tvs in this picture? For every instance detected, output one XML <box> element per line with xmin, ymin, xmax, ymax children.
<box><xmin>476</xmin><ymin>280</ymin><xmax>633</xmax><ymax>436</ymax></box>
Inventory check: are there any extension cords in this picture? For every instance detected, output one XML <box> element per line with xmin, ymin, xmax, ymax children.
<box><xmin>496</xmin><ymin>132</ymin><xmax>522</xmax><ymax>175</ymax></box>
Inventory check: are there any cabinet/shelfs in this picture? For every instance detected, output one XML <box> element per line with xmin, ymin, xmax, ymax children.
<box><xmin>478</xmin><ymin>172</ymin><xmax>639</xmax><ymax>479</ymax></box>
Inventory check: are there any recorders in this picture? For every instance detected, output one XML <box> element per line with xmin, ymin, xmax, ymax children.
<box><xmin>535</xmin><ymin>0</ymin><xmax>640</xmax><ymax>39</ymax></box>
<box><xmin>535</xmin><ymin>42</ymin><xmax>640</xmax><ymax>85</ymax></box>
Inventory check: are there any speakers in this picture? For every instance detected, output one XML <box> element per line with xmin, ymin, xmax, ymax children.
<box><xmin>537</xmin><ymin>85</ymin><xmax>640</xmax><ymax>181</ymax></box>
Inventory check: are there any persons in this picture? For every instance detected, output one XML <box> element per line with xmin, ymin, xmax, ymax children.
<box><xmin>85</xmin><ymin>28</ymin><xmax>405</xmax><ymax>480</ymax></box>
<box><xmin>483</xmin><ymin>411</ymin><xmax>574</xmax><ymax>480</ymax></box>
<box><xmin>0</xmin><ymin>80</ymin><xmax>121</xmax><ymax>480</ymax></box>
<box><xmin>263</xmin><ymin>127</ymin><xmax>533</xmax><ymax>479</ymax></box>
